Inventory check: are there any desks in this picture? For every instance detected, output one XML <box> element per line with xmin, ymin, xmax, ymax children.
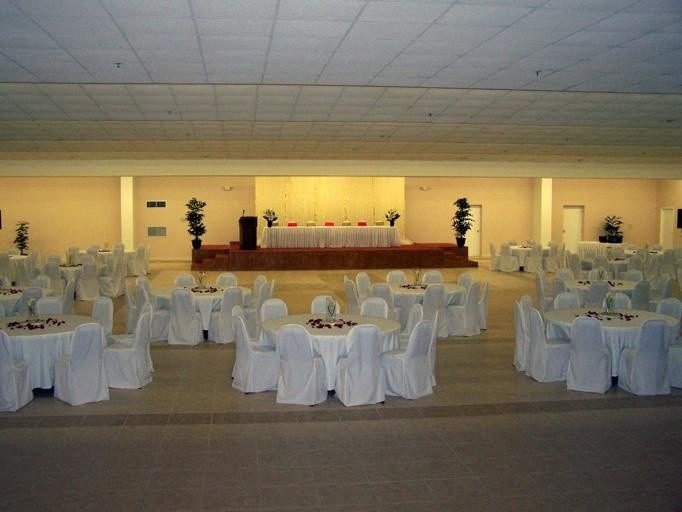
<box><xmin>261</xmin><ymin>226</ymin><xmax>398</xmax><ymax>248</ymax></box>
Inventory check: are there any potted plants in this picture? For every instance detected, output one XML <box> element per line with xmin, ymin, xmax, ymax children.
<box><xmin>12</xmin><ymin>220</ymin><xmax>31</xmax><ymax>255</ymax></box>
<box><xmin>183</xmin><ymin>196</ymin><xmax>207</xmax><ymax>247</ymax></box>
<box><xmin>452</xmin><ymin>197</ymin><xmax>473</xmax><ymax>246</ymax></box>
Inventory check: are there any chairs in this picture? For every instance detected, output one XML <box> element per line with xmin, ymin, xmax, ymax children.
<box><xmin>325</xmin><ymin>221</ymin><xmax>333</xmax><ymax>226</ymax></box>
<box><xmin>375</xmin><ymin>221</ymin><xmax>383</xmax><ymax>225</ymax></box>
<box><xmin>342</xmin><ymin>221</ymin><xmax>351</xmax><ymax>226</ymax></box>
<box><xmin>358</xmin><ymin>221</ymin><xmax>367</xmax><ymax>226</ymax></box>
<box><xmin>288</xmin><ymin>220</ymin><xmax>297</xmax><ymax>226</ymax></box>
<box><xmin>307</xmin><ymin>221</ymin><xmax>317</xmax><ymax>226</ymax></box>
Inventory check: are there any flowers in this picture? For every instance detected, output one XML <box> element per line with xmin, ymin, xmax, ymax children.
<box><xmin>263</xmin><ymin>208</ymin><xmax>278</xmax><ymax>220</ymax></box>
<box><xmin>384</xmin><ymin>208</ymin><xmax>401</xmax><ymax>221</ymax></box>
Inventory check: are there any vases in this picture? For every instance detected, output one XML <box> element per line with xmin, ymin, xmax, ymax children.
<box><xmin>267</xmin><ymin>220</ymin><xmax>276</xmax><ymax>226</ymax></box>
<box><xmin>390</xmin><ymin>219</ymin><xmax>395</xmax><ymax>226</ymax></box>
